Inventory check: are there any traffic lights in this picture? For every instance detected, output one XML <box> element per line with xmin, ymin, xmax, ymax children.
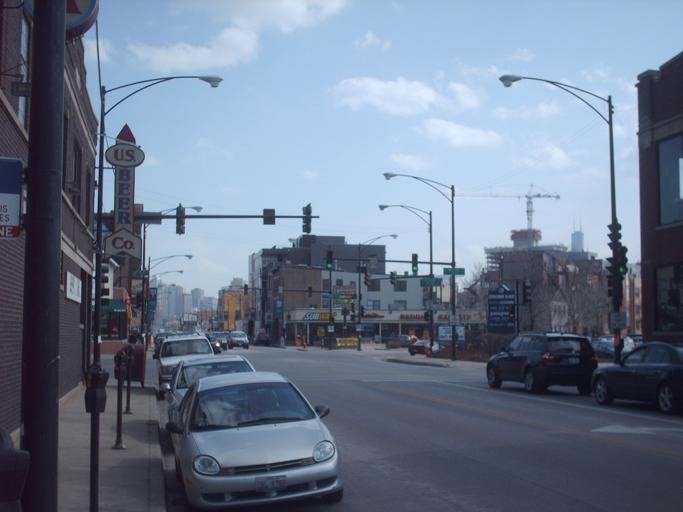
<box><xmin>326</xmin><ymin>251</ymin><xmax>334</xmax><ymax>270</ymax></box>
<box><xmin>412</xmin><ymin>254</ymin><xmax>418</xmax><ymax>272</ymax></box>
<box><xmin>606</xmin><ymin>224</ymin><xmax>627</xmax><ymax>297</ymax></box>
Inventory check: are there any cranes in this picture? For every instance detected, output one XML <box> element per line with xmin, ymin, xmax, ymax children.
<box><xmin>455</xmin><ymin>184</ymin><xmax>560</xmax><ymax>247</ymax></box>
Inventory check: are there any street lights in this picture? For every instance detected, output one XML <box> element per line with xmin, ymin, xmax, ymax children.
<box><xmin>384</xmin><ymin>173</ymin><xmax>456</xmax><ymax>361</ymax></box>
<box><xmin>379</xmin><ymin>205</ymin><xmax>433</xmax><ymax>352</ymax></box>
<box><xmin>85</xmin><ymin>76</ymin><xmax>223</xmax><ymax>512</ymax></box>
<box><xmin>140</xmin><ymin>207</ymin><xmax>202</xmax><ymax>334</ymax></box>
<box><xmin>357</xmin><ymin>235</ymin><xmax>397</xmax><ymax>351</ymax></box>
<box><xmin>499</xmin><ymin>76</ymin><xmax>620</xmax><ymax>363</ymax></box>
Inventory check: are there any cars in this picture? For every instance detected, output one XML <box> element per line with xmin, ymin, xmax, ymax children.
<box><xmin>166</xmin><ymin>371</ymin><xmax>343</xmax><ymax>512</ymax></box>
<box><xmin>591</xmin><ymin>341</ymin><xmax>683</xmax><ymax>413</ymax></box>
<box><xmin>153</xmin><ymin>330</ymin><xmax>256</xmax><ymax>419</ymax></box>
<box><xmin>408</xmin><ymin>340</ymin><xmax>431</xmax><ymax>355</ymax></box>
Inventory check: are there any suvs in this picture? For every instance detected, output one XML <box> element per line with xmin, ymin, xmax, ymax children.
<box><xmin>487</xmin><ymin>333</ymin><xmax>598</xmax><ymax>395</ymax></box>
